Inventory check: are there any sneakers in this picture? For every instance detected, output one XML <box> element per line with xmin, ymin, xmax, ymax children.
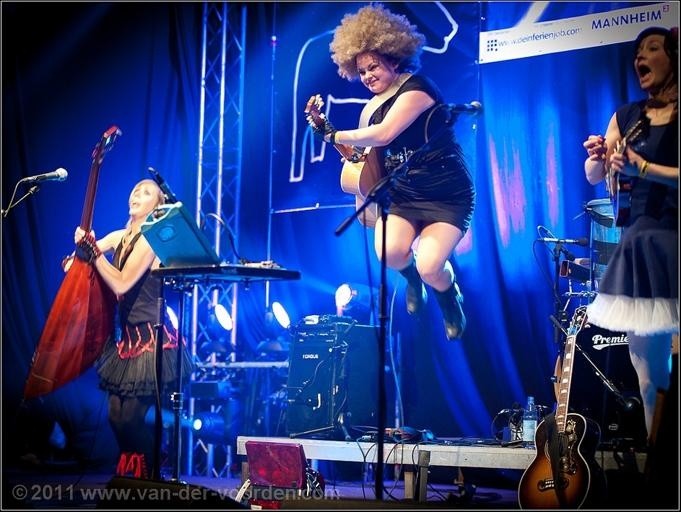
<box><xmin>116</xmin><ymin>443</ymin><xmax>156</xmax><ymax>483</ymax></box>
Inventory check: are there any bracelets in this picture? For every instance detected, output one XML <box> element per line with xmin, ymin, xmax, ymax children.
<box><xmin>640</xmin><ymin>159</ymin><xmax>650</xmax><ymax>177</ymax></box>
<box><xmin>329</xmin><ymin>129</ymin><xmax>337</xmax><ymax>144</ymax></box>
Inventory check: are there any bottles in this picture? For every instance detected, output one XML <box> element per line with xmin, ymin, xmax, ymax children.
<box><xmin>522</xmin><ymin>396</ymin><xmax>537</xmax><ymax>449</ymax></box>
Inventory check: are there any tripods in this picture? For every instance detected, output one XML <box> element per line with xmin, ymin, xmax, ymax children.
<box><xmin>289</xmin><ymin>320</ymin><xmax>367</xmax><ymax>441</ymax></box>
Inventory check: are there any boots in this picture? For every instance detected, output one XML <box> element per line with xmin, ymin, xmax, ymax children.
<box><xmin>399</xmin><ymin>264</ymin><xmax>428</xmax><ymax>318</ymax></box>
<box><xmin>433</xmin><ymin>283</ymin><xmax>465</xmax><ymax>342</ymax></box>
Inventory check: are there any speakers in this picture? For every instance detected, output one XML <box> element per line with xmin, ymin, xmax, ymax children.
<box><xmin>96</xmin><ymin>477</ymin><xmax>250</xmax><ymax>509</ymax></box>
<box><xmin>568</xmin><ymin>324</ymin><xmax>649</xmax><ymax>452</ymax></box>
<box><xmin>285</xmin><ymin>324</ymin><xmax>380</xmax><ymax>437</ymax></box>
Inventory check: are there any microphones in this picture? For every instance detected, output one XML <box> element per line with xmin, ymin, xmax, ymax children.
<box><xmin>537</xmin><ymin>236</ymin><xmax>589</xmax><ymax>247</ymax></box>
<box><xmin>287</xmin><ymin>397</ymin><xmax>319</xmax><ymax>407</ymax></box>
<box><xmin>440</xmin><ymin>100</ymin><xmax>484</xmax><ymax>116</ymax></box>
<box><xmin>21</xmin><ymin>166</ymin><xmax>70</xmax><ymax>185</ymax></box>
<box><xmin>155</xmin><ymin>172</ymin><xmax>177</xmax><ymax>204</ymax></box>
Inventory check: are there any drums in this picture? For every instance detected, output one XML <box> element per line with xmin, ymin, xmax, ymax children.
<box><xmin>587</xmin><ymin>198</ymin><xmax>624</xmax><ymax>305</ymax></box>
<box><xmin>551</xmin><ymin>317</ymin><xmax>680</xmax><ymax>451</ymax></box>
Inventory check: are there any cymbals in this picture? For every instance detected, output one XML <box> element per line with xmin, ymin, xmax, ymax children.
<box><xmin>575</xmin><ymin>258</ymin><xmax>599</xmax><ymax>272</ymax></box>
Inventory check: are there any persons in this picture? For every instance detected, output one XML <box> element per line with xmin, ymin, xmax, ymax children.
<box><xmin>305</xmin><ymin>2</ymin><xmax>476</xmax><ymax>342</ymax></box>
<box><xmin>582</xmin><ymin>26</ymin><xmax>681</xmax><ymax>447</ymax></box>
<box><xmin>62</xmin><ymin>178</ymin><xmax>196</xmax><ymax>487</ymax></box>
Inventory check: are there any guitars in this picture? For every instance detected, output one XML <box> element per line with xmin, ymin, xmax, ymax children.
<box><xmin>518</xmin><ymin>304</ymin><xmax>604</xmax><ymax>509</ymax></box>
<box><xmin>606</xmin><ymin>112</ymin><xmax>651</xmax><ymax>227</ymax></box>
<box><xmin>304</xmin><ymin>94</ymin><xmax>383</xmax><ymax>227</ymax></box>
